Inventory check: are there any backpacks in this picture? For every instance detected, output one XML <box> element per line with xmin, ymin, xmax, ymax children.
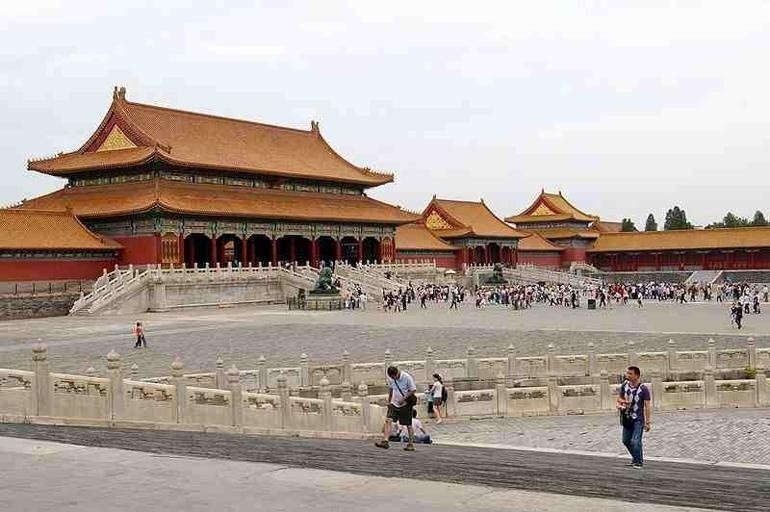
<box><xmin>442</xmin><ymin>385</ymin><xmax>448</xmax><ymax>400</ymax></box>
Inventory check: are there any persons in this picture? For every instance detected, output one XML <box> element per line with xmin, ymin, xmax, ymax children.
<box><xmin>716</xmin><ymin>280</ymin><xmax>769</xmax><ymax>329</ymax></box>
<box><xmin>381</xmin><ymin>373</ymin><xmax>448</xmax><ymax>444</ymax></box>
<box><xmin>617</xmin><ymin>365</ymin><xmax>651</xmax><ymax>469</ymax></box>
<box><xmin>582</xmin><ymin>279</ymin><xmax>712</xmax><ymax>308</ymax></box>
<box><xmin>132</xmin><ymin>322</ymin><xmax>142</xmax><ymax>349</ymax></box>
<box><xmin>616</xmin><ymin>377</ymin><xmax>630</xmax><ymax>409</ymax></box>
<box><xmin>373</xmin><ymin>366</ymin><xmax>418</xmax><ymax>451</ymax></box>
<box><xmin>334</xmin><ymin>277</ymin><xmax>368</xmax><ymax>310</ymax></box>
<box><xmin>380</xmin><ymin>280</ymin><xmax>464</xmax><ymax>313</ymax></box>
<box><xmin>134</xmin><ymin>320</ymin><xmax>147</xmax><ymax>347</ymax></box>
<box><xmin>472</xmin><ymin>283</ymin><xmax>581</xmax><ymax>310</ymax></box>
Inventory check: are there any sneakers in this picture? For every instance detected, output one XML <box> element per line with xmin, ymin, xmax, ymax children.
<box><xmin>435</xmin><ymin>418</ymin><xmax>442</xmax><ymax>424</ymax></box>
<box><xmin>375</xmin><ymin>440</ymin><xmax>389</xmax><ymax>449</ymax></box>
<box><xmin>626</xmin><ymin>462</ymin><xmax>644</xmax><ymax>469</ymax></box>
<box><xmin>404</xmin><ymin>443</ymin><xmax>415</xmax><ymax>451</ymax></box>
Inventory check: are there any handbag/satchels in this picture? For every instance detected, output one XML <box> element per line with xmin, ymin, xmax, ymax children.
<box><xmin>620</xmin><ymin>414</ymin><xmax>634</xmax><ymax>429</ymax></box>
<box><xmin>405</xmin><ymin>395</ymin><xmax>417</xmax><ymax>406</ymax></box>
